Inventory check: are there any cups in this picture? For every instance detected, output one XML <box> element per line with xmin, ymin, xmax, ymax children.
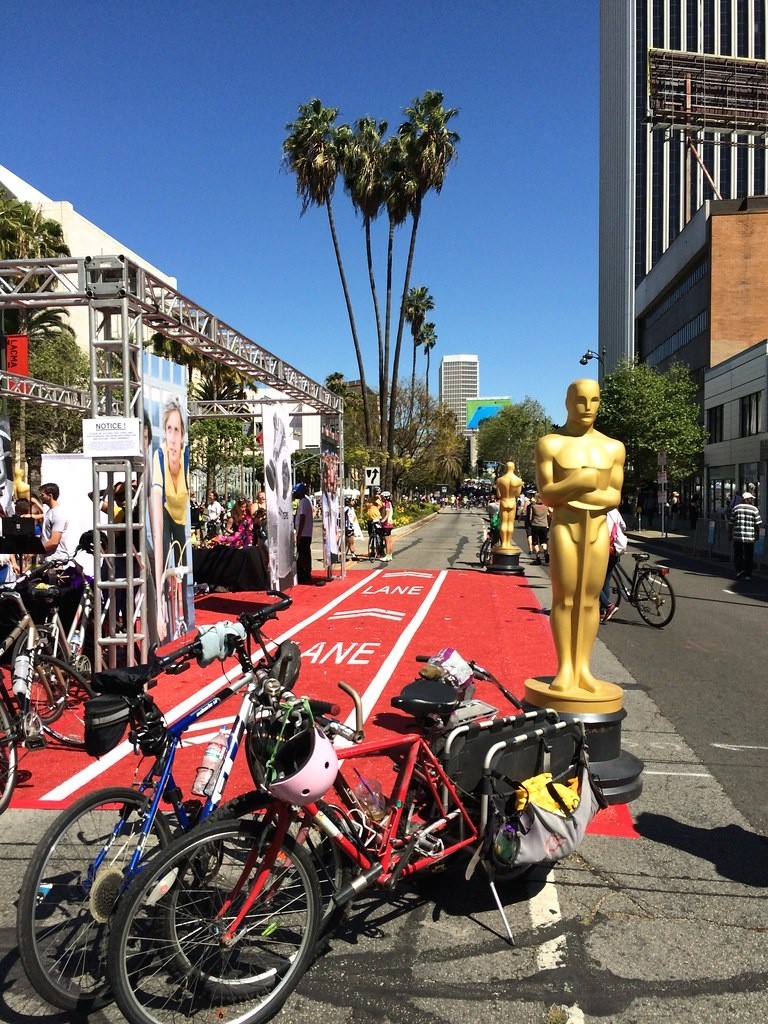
<box><xmin>354</xmin><ymin>780</ymin><xmax>386</xmax><ymax>820</ymax></box>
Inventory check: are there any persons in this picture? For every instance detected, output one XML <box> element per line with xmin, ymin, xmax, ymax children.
<box><xmin>150</xmin><ymin>395</ymin><xmax>191</xmax><ymax>637</ymax></box>
<box><xmin>6</xmin><ymin>471</ymin><xmax>138</xmax><ymax>645</ymax></box>
<box><xmin>292</xmin><ymin>483</ymin><xmax>314</xmax><ymax>583</ymax></box>
<box><xmin>143</xmin><ymin>406</ymin><xmax>163</xmax><ymax>643</ymax></box>
<box><xmin>12</xmin><ymin>468</ymin><xmax>31</xmax><ymax>502</ymax></box>
<box><xmin>271</xmin><ymin>459</ymin><xmax>297</xmax><ymax>592</ymax></box>
<box><xmin>534</xmin><ymin>378</ymin><xmax>626</xmax><ymax>694</ymax></box>
<box><xmin>517</xmin><ymin>485</ymin><xmax>553</xmax><ymax>567</ymax></box>
<box><xmin>638</xmin><ymin>489</ymin><xmax>706</xmax><ymax>533</ymax></box>
<box><xmin>729</xmin><ymin>491</ymin><xmax>743</xmax><ymax>508</ymax></box>
<box><xmin>338</xmin><ymin>485</ymin><xmax>395</xmax><ymax>564</ymax></box>
<box><xmin>727</xmin><ymin>492</ymin><xmax>763</xmax><ymax>580</ymax></box>
<box><xmin>599</xmin><ymin>508</ymin><xmax>629</xmax><ymax>621</ymax></box>
<box><xmin>289</xmin><ymin>495</ymin><xmax>323</xmax><ymax>523</ymax></box>
<box><xmin>402</xmin><ymin>485</ymin><xmax>495</xmax><ymax>510</ymax></box>
<box><xmin>191</xmin><ymin>482</ymin><xmax>268</xmax><ymax>564</ymax></box>
<box><xmin>496</xmin><ymin>461</ymin><xmax>522</xmax><ymax>548</ymax></box>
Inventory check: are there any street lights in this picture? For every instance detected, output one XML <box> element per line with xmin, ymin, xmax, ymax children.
<box><xmin>580</xmin><ymin>348</ymin><xmax>606</xmax><ymax>392</ymax></box>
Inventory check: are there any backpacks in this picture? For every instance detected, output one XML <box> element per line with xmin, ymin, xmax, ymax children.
<box><xmin>490</xmin><ymin>511</ymin><xmax>501</xmax><ymax>531</ymax></box>
<box><xmin>610</xmin><ymin>520</ymin><xmax>628</xmax><ymax>556</ymax></box>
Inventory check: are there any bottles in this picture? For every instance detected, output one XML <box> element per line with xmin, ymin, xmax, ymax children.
<box><xmin>191</xmin><ymin>725</ymin><xmax>229</xmax><ymax>795</ymax></box>
<box><xmin>70</xmin><ymin>630</ymin><xmax>80</xmax><ymax>653</ymax></box>
<box><xmin>12</xmin><ymin>650</ymin><xmax>29</xmax><ymax>694</ymax></box>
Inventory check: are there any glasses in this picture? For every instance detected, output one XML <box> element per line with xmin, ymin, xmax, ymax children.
<box><xmin>240</xmin><ymin>498</ymin><xmax>247</xmax><ymax>501</ymax></box>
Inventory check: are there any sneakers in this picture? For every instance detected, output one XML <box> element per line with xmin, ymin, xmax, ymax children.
<box><xmin>529</xmin><ymin>550</ymin><xmax>533</xmax><ymax>554</ymax></box>
<box><xmin>379</xmin><ymin>555</ymin><xmax>392</xmax><ymax>561</ymax></box>
<box><xmin>530</xmin><ymin>557</ymin><xmax>542</xmax><ymax>565</ymax></box>
<box><xmin>544</xmin><ymin>554</ymin><xmax>550</xmax><ymax>566</ymax></box>
<box><xmin>600</xmin><ymin>605</ymin><xmax>618</xmax><ymax>624</ymax></box>
<box><xmin>352</xmin><ymin>557</ymin><xmax>357</xmax><ymax>560</ymax></box>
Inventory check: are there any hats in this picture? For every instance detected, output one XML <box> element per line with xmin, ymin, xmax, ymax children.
<box><xmin>742</xmin><ymin>492</ymin><xmax>755</xmax><ymax>500</ymax></box>
<box><xmin>293</xmin><ymin>483</ymin><xmax>307</xmax><ymax>493</ymax></box>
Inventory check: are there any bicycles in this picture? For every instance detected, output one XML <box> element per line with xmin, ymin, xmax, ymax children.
<box><xmin>0</xmin><ymin>562</ymin><xmax>607</xmax><ymax>1024</ymax></box>
<box><xmin>607</xmin><ymin>553</ymin><xmax>675</xmax><ymax>628</ymax></box>
<box><xmin>476</xmin><ymin>518</ymin><xmax>502</xmax><ymax>563</ymax></box>
<box><xmin>367</xmin><ymin>521</ymin><xmax>386</xmax><ymax>562</ymax></box>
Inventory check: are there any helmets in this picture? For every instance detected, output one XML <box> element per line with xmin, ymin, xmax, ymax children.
<box><xmin>78</xmin><ymin>529</ymin><xmax>108</xmax><ymax>554</ymax></box>
<box><xmin>245</xmin><ymin>714</ymin><xmax>338</xmax><ymax>801</ymax></box>
<box><xmin>381</xmin><ymin>491</ymin><xmax>393</xmax><ymax>501</ymax></box>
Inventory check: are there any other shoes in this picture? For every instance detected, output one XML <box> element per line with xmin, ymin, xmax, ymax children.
<box><xmin>735</xmin><ymin>569</ymin><xmax>745</xmax><ymax>578</ymax></box>
<box><xmin>745</xmin><ymin>576</ymin><xmax>752</xmax><ymax>580</ymax></box>
